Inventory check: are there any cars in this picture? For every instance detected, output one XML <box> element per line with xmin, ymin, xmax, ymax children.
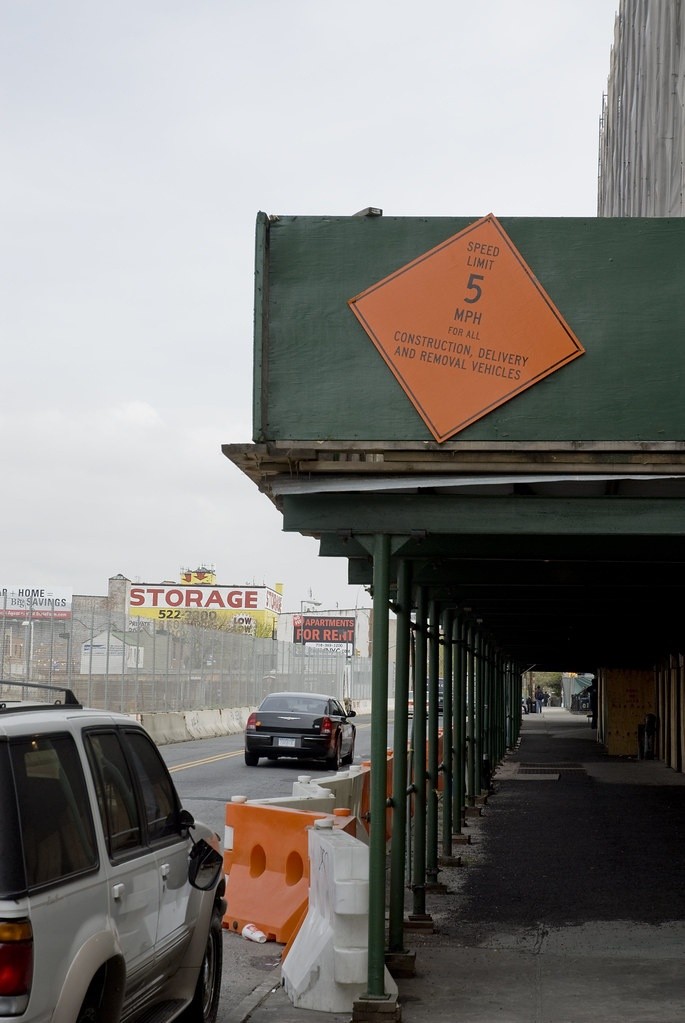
<box><xmin>245</xmin><ymin>691</ymin><xmax>357</xmax><ymax>771</ymax></box>
<box><xmin>408</xmin><ymin>670</ymin><xmax>476</xmax><ymax>719</ymax></box>
<box><xmin>0</xmin><ymin>679</ymin><xmax>227</xmax><ymax>1022</ymax></box>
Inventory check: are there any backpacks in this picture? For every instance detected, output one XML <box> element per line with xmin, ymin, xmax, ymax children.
<box><xmin>537</xmin><ymin>692</ymin><xmax>543</xmax><ymax>700</ymax></box>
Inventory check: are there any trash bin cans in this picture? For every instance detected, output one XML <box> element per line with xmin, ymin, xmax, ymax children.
<box><xmin>532</xmin><ymin>700</ymin><xmax>540</xmax><ymax>713</ymax></box>
<box><xmin>635</xmin><ymin>713</ymin><xmax>659</xmax><ymax>760</ymax></box>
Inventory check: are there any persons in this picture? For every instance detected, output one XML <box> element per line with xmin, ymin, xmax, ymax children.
<box><xmin>535</xmin><ymin>685</ymin><xmax>543</xmax><ymax>713</ymax></box>
<box><xmin>543</xmin><ymin>692</ymin><xmax>550</xmax><ymax>706</ymax></box>
